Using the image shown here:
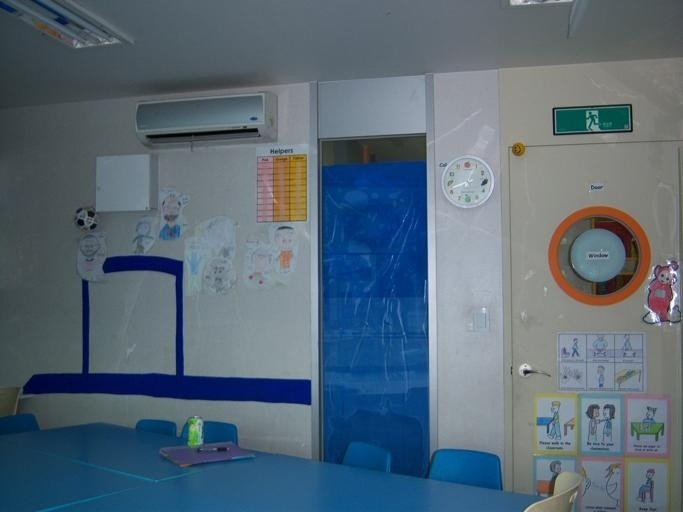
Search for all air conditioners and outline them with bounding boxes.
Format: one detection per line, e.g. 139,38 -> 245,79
134,92 -> 278,153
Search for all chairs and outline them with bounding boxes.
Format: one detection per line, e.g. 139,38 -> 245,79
524,471 -> 583,512
428,447 -> 504,491
341,442 -> 391,473
0,413 -> 41,436
136,418 -> 176,436
181,420 -> 239,447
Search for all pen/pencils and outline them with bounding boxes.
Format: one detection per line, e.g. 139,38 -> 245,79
197,447 -> 231,452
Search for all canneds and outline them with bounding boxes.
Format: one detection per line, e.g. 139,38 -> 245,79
188,416 -> 204,447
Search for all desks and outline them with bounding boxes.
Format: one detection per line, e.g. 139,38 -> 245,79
0,422 -> 549,512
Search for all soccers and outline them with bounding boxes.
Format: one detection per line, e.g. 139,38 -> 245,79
74,206 -> 100,234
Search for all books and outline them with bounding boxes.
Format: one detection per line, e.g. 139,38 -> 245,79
159,441 -> 257,468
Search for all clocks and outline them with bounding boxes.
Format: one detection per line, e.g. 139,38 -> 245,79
441,155 -> 495,209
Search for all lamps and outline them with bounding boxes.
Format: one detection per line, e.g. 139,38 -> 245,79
0,0 -> 136,49
